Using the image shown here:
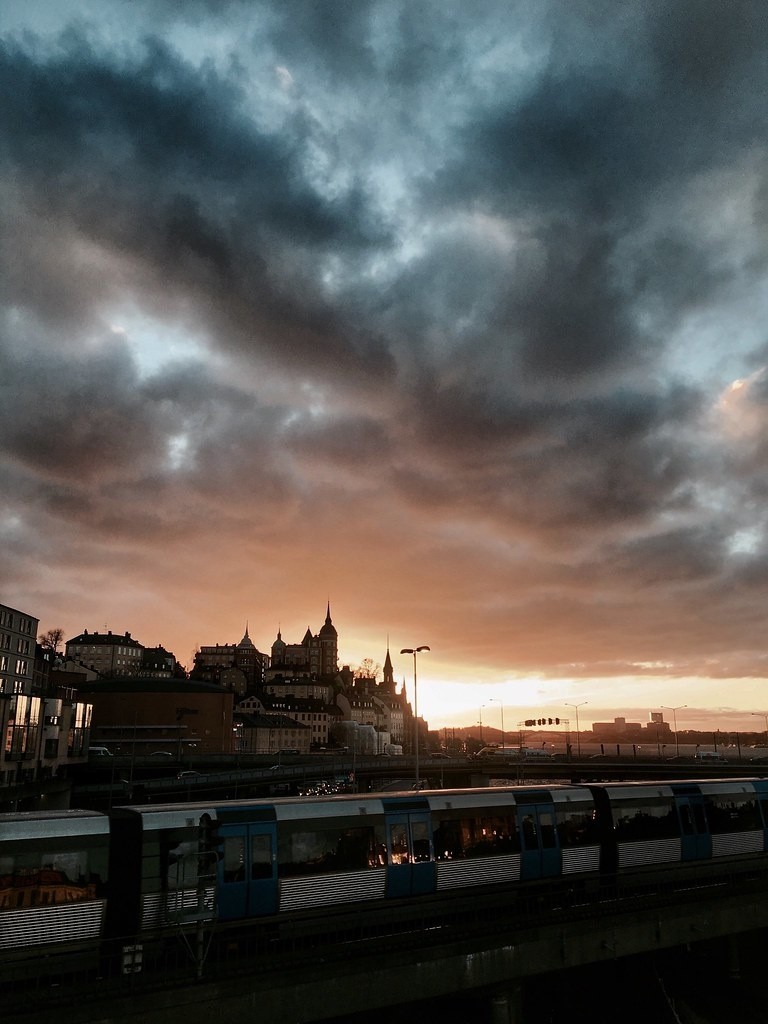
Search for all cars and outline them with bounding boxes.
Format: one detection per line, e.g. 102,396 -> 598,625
750,756 -> 768,763
430,753 -> 450,758
590,753 -> 606,758
177,771 -> 206,779
298,781 -> 352,796
666,756 -> 689,763
151,750 -> 172,756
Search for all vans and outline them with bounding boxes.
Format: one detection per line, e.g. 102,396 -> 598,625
477,747 -> 515,755
273,749 -> 299,755
89,746 -> 113,756
697,751 -> 728,765
525,750 -> 551,757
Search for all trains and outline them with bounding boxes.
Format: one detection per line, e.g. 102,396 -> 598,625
0,777 -> 768,969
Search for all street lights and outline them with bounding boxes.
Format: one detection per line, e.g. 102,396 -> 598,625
401,646 -> 430,790
660,704 -> 687,756
565,702 -> 588,757
490,698 -> 505,760
479,704 -> 486,742
